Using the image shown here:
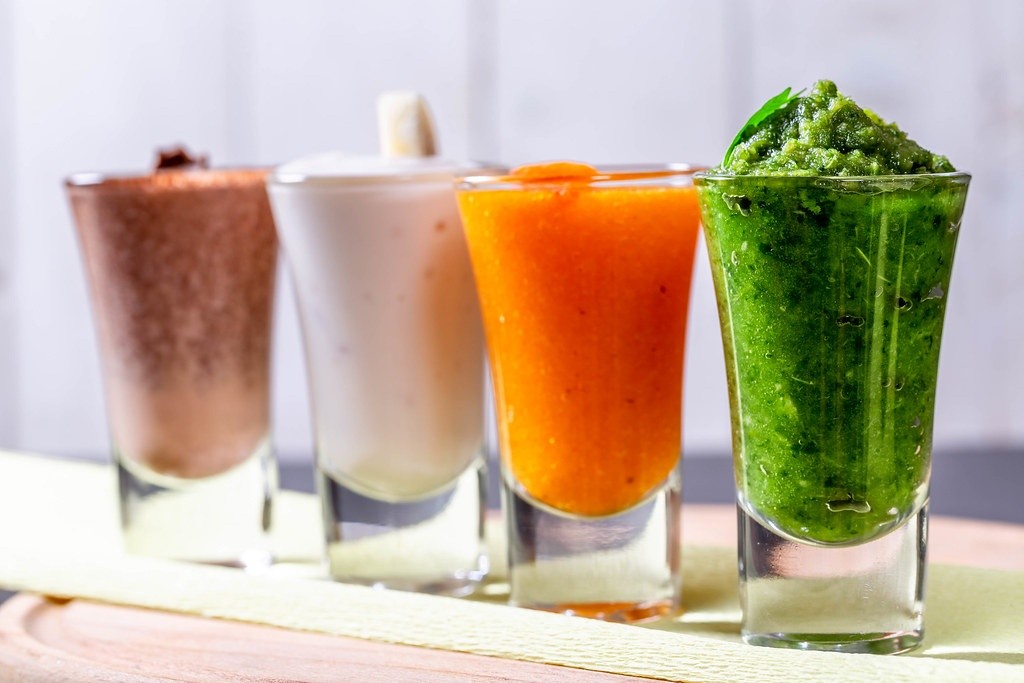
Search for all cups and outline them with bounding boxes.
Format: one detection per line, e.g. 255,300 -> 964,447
62,165 -> 279,564
453,164 -> 707,624
693,165 -> 973,656
265,163 -> 510,596
